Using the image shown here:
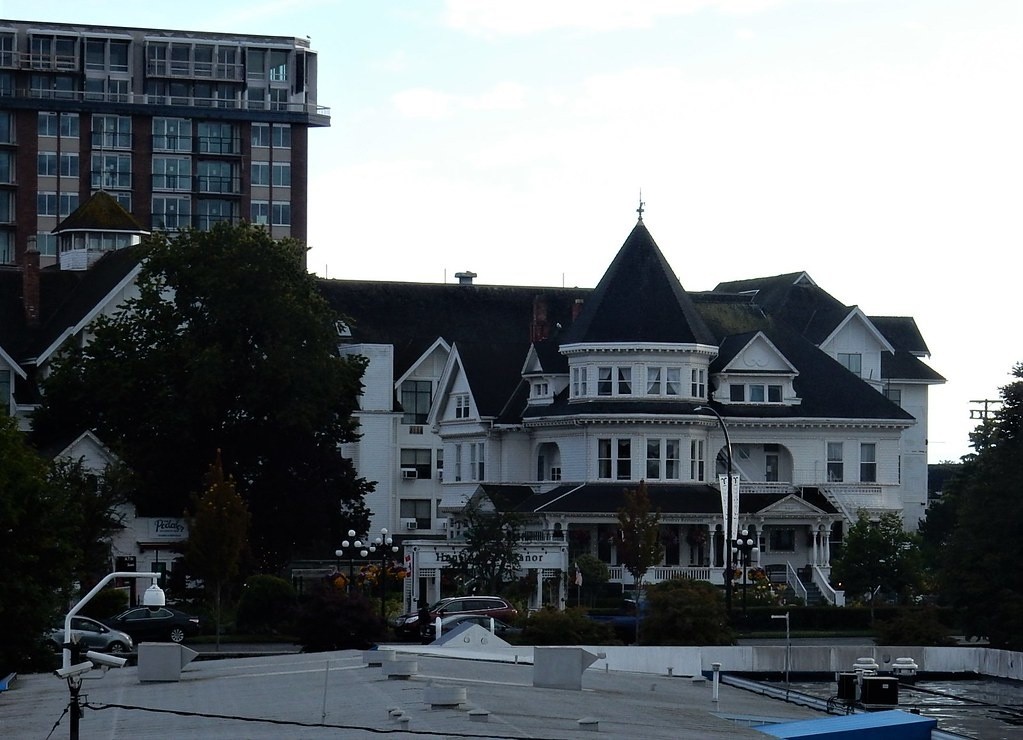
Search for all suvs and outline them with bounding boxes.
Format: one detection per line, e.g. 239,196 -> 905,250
394,596 -> 520,639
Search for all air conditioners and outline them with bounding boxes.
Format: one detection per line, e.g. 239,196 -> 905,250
401,469 -> 418,479
406,521 -> 417,530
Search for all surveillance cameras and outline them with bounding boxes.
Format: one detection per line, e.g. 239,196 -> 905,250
144,588 -> 166,613
53,661 -> 93,680
86,651 -> 128,669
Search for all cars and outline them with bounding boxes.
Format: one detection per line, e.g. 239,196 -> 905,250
104,606 -> 201,644
44,614 -> 134,655
421,614 -> 525,645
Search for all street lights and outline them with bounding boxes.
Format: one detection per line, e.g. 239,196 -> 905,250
62,571 -> 166,740
336,530 -> 370,593
367,528 -> 399,625
737,529 -> 754,621
694,406 -> 733,617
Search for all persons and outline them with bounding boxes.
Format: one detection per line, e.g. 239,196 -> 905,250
368,639 -> 381,667
69,632 -> 80,667
417,602 -> 432,642
805,561 -> 812,583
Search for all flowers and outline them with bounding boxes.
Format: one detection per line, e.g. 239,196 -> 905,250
662,529 -> 680,545
355,563 -> 386,587
388,563 -> 411,583
686,528 -> 708,546
722,568 -> 742,580
322,570 -> 350,589
748,567 -> 765,581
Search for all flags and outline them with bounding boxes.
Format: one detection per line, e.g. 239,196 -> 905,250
575,563 -> 582,586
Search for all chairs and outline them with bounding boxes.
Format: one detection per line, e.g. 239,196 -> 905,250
798,564 -> 812,582
